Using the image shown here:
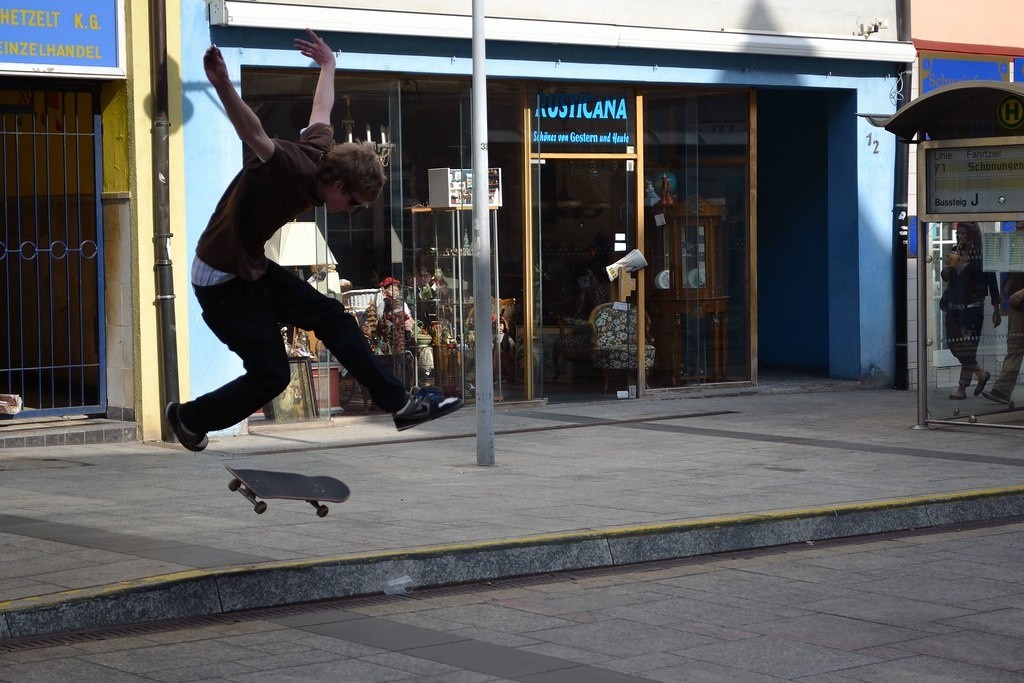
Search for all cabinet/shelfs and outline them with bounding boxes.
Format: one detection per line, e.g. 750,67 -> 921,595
403,203 -> 505,405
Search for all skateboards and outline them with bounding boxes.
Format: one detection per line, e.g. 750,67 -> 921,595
223,463 -> 351,517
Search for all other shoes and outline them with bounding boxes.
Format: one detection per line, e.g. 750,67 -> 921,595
939,288 -> 948,312
974,371 -> 991,395
949,392 -> 967,400
982,390 -> 1011,403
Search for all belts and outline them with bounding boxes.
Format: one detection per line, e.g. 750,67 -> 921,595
948,302 -> 983,310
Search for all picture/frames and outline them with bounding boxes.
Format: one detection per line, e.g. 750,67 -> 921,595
267,356 -> 320,425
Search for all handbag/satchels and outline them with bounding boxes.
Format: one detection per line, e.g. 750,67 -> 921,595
1002,272 -> 1024,297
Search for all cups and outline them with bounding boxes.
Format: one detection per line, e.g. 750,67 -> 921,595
948,250 -> 960,268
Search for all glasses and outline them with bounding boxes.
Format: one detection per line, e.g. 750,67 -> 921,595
347,193 -> 364,208
956,231 -> 967,235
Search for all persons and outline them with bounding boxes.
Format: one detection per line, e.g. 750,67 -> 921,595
982,221 -> 1024,404
377,277 -> 412,350
163,28 -> 464,452
939,221 -> 1001,399
405,261 -> 438,326
491,316 -> 517,384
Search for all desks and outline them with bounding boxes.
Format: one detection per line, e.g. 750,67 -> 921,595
515,322 -> 591,385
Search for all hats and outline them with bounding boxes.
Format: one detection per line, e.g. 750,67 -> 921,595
379,277 -> 400,290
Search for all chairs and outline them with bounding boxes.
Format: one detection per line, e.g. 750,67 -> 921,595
587,301 -> 657,392
456,298 -> 518,381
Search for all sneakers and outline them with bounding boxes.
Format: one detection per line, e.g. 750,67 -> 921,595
163,401 -> 209,452
392,390 -> 465,432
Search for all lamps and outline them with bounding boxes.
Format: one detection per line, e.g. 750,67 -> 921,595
263,217 -> 338,354
